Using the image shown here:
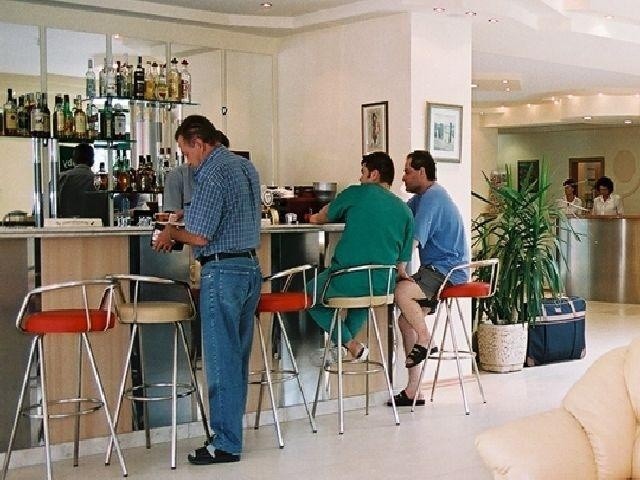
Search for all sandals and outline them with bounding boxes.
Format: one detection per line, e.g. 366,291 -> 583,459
188,444 -> 242,465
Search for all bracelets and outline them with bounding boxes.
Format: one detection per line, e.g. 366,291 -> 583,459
170,225 -> 180,245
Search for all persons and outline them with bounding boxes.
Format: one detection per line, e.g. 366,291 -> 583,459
302,150 -> 416,367
57,144 -> 109,219
152,110 -> 263,466
555,179 -> 584,218
590,176 -> 624,217
161,129 -> 233,216
387,150 -> 471,408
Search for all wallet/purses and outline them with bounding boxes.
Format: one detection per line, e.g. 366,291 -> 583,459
150,222 -> 185,251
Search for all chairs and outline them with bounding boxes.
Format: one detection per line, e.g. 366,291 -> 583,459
1,275 -> 133,480
102,273 -> 209,470
308,261 -> 406,435
246,262 -> 318,450
411,258 -> 500,416
470,337 -> 639,479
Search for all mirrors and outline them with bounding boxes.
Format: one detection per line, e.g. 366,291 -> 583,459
0,22 -> 41,229
42,28 -> 109,221
225,51 -> 276,185
109,34 -> 169,229
169,43 -> 225,172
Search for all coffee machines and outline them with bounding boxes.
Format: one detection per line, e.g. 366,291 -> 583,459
312,182 -> 336,206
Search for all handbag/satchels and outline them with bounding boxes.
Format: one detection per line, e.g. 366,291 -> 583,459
524,296 -> 586,367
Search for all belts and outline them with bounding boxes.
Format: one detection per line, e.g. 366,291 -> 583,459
195,248 -> 257,266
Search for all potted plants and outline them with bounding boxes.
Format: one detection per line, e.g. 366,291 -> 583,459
468,153 -> 597,373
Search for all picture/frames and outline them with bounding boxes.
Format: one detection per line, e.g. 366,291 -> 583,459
361,99 -> 389,158
424,101 -> 463,165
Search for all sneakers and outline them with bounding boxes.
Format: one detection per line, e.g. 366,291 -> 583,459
352,342 -> 370,363
310,345 -> 347,367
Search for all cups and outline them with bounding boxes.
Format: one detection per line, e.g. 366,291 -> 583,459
138,218 -> 150,230
117,217 -> 127,228
94,175 -> 102,191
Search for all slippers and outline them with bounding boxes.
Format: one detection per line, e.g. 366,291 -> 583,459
406,342 -> 438,367
388,390 -> 425,406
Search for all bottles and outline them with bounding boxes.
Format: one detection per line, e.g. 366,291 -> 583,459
97,148 -> 175,192
123,198 -> 132,228
4,88 -> 128,139
84,54 -> 193,102
